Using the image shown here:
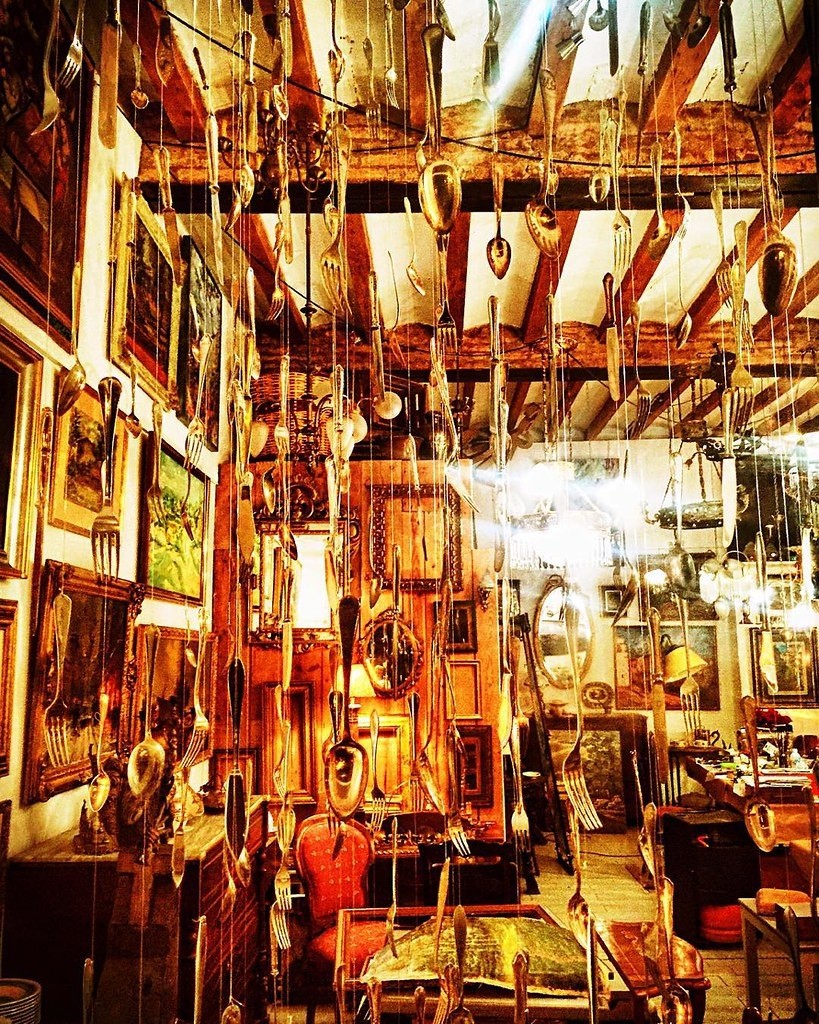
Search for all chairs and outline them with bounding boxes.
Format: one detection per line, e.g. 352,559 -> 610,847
736,786 -> 819,1024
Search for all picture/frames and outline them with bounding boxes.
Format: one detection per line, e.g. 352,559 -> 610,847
18,558 -> 138,805
611,621 -> 721,714
0,0 -> 97,355
534,716 -> 637,830
136,429 -> 211,606
433,600 -> 479,654
749,626 -> 819,710
109,180 -> 185,412
179,235 -> 225,452
247,518 -> 344,642
0,317 -> 44,581
47,367 -> 133,547
370,483 -> 464,595
135,623 -> 215,770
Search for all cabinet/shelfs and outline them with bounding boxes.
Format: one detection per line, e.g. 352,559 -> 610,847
6,794 -> 269,1023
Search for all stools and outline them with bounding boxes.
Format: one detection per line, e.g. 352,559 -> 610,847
596,918 -> 712,1024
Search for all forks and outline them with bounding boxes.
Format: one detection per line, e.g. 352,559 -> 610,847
0,1 -> 818,1024
